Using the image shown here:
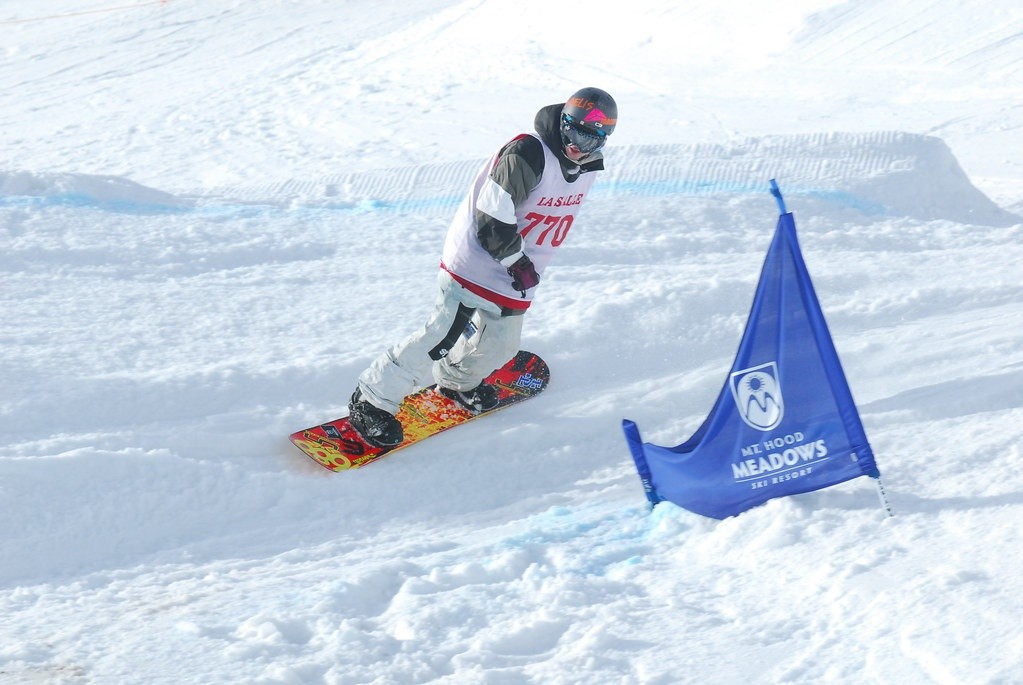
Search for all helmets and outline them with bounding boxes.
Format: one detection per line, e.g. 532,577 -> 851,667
559,87 -> 617,138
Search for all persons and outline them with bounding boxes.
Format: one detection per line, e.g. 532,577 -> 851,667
347,87 -> 618,447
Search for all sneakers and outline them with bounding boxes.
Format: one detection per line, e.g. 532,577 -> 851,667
348,386 -> 403,448
439,378 -> 498,410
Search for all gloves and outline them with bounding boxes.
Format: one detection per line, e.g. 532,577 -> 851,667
507,254 -> 539,292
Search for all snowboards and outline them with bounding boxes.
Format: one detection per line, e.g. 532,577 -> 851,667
289,349 -> 551,475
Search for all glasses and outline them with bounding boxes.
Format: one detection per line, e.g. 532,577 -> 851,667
561,125 -> 603,153
